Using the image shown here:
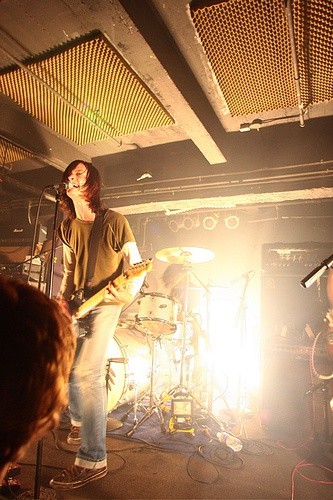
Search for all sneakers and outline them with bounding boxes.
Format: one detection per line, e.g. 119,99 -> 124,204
49,465 -> 108,490
67,425 -> 81,444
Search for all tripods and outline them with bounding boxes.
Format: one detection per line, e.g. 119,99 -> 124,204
118,262 -> 225,444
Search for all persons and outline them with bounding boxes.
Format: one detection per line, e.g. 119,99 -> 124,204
326,267 -> 333,360
49,160 -> 147,491
161,264 -> 200,399
0,277 -> 70,500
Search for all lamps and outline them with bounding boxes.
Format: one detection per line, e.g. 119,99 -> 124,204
172,399 -> 193,416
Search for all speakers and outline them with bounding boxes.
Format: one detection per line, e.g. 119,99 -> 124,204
259,242 -> 333,445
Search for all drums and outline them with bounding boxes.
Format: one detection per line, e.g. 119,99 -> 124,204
136,292 -> 181,336
167,321 -> 194,342
103,318 -> 160,414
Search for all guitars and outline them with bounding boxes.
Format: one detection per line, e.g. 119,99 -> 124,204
61,258 -> 154,340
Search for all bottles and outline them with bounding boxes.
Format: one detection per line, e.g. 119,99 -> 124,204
216,432 -> 243,451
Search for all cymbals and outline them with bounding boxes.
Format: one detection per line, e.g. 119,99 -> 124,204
188,285 -> 231,292
155,246 -> 216,264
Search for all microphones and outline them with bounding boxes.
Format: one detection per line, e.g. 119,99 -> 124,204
304,380 -> 329,397
230,270 -> 254,284
46,182 -> 72,190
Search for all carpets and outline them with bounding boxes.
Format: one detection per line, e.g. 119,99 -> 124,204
106,404 -> 228,458
